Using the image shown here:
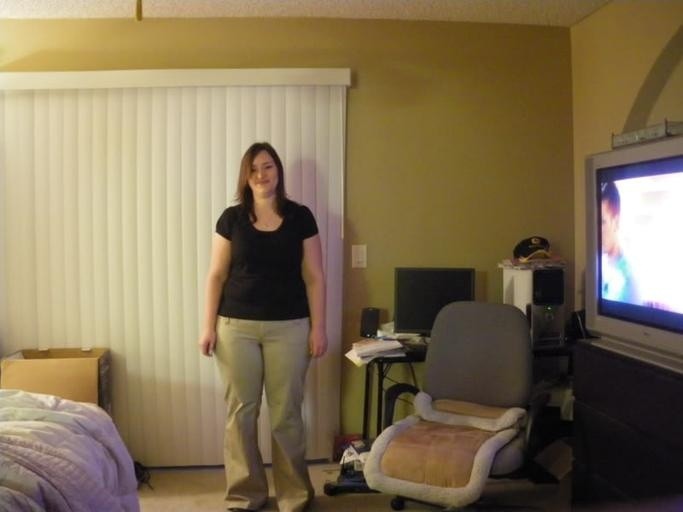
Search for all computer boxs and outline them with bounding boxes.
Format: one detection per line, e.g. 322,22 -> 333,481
502,264 -> 565,350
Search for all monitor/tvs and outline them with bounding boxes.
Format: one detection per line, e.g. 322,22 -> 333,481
393,267 -> 475,345
586,135 -> 682,376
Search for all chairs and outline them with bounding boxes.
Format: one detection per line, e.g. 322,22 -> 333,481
360,301 -> 533,511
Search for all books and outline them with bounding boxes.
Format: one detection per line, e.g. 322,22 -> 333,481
343,338 -> 407,368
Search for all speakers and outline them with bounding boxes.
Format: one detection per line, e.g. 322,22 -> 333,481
359,307 -> 380,338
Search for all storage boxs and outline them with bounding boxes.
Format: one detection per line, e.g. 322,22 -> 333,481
0,345 -> 112,414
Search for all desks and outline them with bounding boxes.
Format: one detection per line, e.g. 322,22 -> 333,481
359,343 -> 576,449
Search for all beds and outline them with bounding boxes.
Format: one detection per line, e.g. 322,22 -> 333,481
0,389 -> 140,512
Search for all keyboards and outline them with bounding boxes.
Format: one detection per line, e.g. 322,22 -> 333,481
405,346 -> 428,354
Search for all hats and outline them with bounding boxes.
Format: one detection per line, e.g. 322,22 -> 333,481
512,236 -> 552,263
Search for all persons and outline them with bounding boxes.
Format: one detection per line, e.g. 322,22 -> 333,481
599,179 -> 644,308
198,141 -> 329,511
